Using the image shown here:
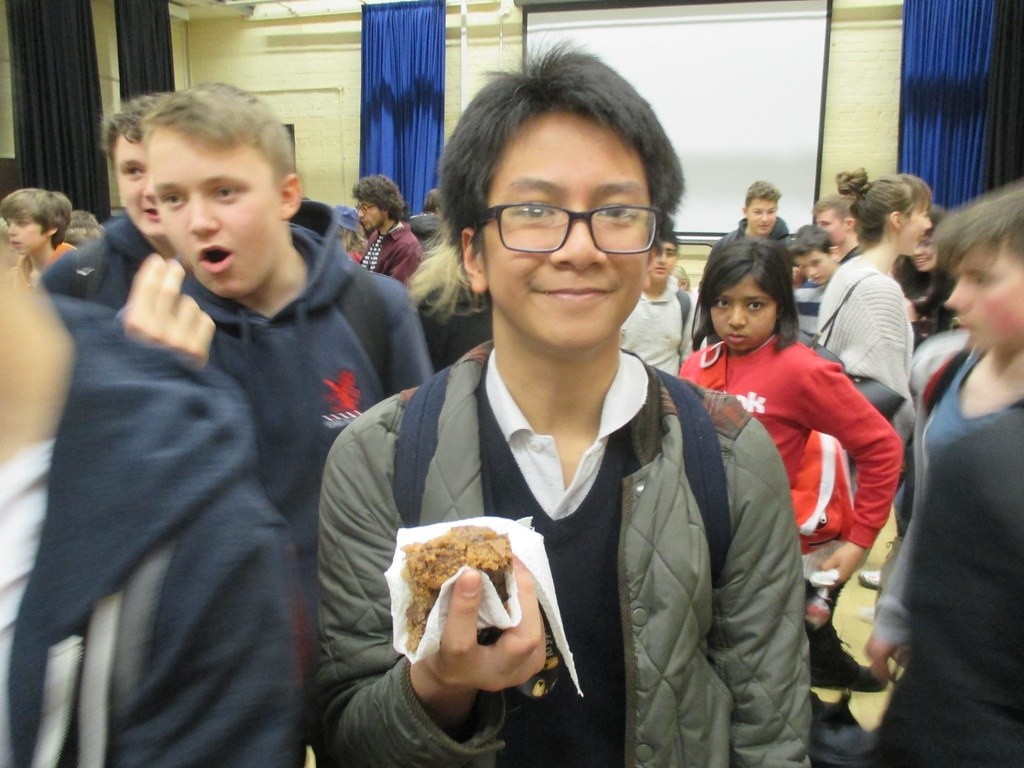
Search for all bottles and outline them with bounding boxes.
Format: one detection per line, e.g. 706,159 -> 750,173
804,585 -> 832,633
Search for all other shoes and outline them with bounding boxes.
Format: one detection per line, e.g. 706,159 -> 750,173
857,569 -> 881,588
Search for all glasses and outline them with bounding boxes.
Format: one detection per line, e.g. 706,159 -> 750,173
469,201 -> 662,254
355,202 -> 376,214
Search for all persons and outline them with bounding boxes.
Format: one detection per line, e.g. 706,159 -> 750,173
0,46 -> 1024,768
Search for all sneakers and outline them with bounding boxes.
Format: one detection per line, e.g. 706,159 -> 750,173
803,618 -> 886,692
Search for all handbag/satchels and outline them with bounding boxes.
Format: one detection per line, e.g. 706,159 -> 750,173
796,272 -> 907,475
